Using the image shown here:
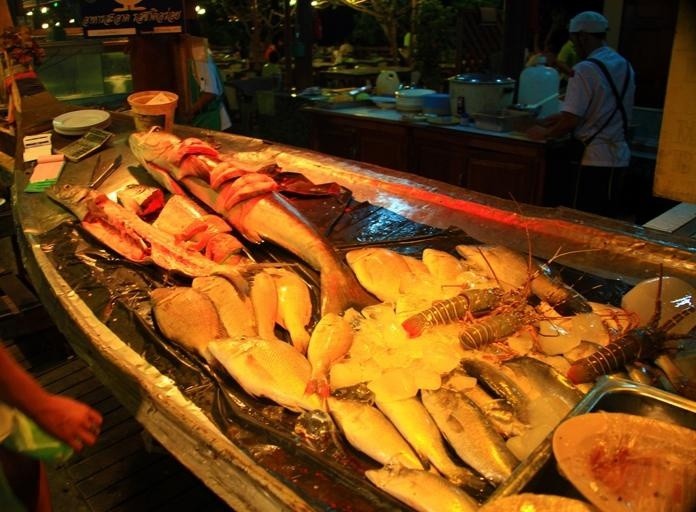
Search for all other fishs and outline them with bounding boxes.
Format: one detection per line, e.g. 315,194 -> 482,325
148,242 -> 690,511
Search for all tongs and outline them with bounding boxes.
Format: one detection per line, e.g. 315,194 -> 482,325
87,151 -> 123,191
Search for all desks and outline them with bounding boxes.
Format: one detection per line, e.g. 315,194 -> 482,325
301,102 -> 574,207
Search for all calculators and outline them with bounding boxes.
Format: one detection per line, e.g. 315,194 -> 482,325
56,127 -> 112,163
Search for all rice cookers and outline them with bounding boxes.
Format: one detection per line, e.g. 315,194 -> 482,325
449,74 -> 517,115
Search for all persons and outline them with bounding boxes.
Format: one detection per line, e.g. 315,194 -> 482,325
0,336 -> 104,512
176,36 -> 232,133
233,11 -> 340,123
523,3 -> 636,215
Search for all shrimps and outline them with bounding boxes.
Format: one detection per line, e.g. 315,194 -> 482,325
402,189 -> 604,357
565,262 -> 696,385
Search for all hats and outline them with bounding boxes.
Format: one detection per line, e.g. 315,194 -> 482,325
569,12 -> 610,35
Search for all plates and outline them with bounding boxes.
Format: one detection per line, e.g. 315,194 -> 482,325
369,94 -> 395,108
421,93 -> 450,115
54,109 -> 113,136
393,88 -> 434,113
